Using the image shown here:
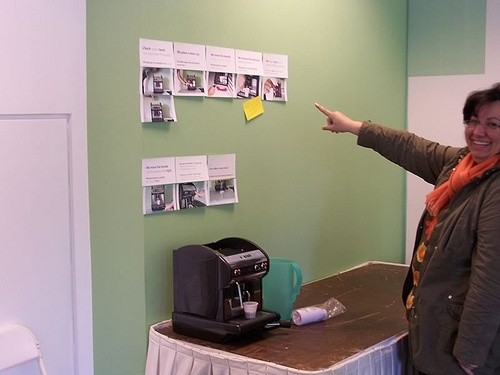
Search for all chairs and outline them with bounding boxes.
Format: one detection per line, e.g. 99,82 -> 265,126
0,324 -> 48,375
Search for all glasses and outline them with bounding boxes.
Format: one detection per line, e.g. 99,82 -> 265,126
463,120 -> 500,129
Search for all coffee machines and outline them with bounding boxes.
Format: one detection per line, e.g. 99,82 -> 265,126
172,237 -> 282,339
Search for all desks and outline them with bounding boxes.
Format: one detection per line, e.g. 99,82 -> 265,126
145,261 -> 410,375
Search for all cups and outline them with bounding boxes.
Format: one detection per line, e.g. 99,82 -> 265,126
243,302 -> 258,319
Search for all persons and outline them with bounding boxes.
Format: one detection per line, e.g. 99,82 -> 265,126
313,82 -> 500,375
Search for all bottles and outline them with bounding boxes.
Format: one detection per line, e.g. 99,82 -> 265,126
292,306 -> 329,326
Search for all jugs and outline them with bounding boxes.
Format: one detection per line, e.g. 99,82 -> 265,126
262,257 -> 303,322
